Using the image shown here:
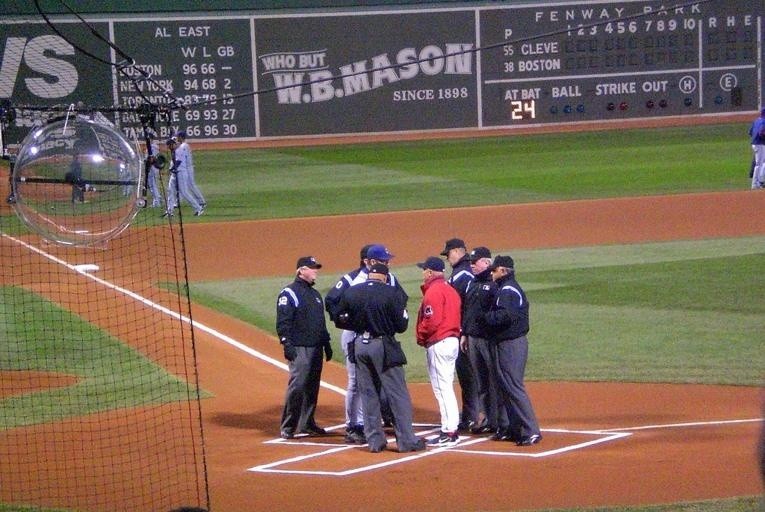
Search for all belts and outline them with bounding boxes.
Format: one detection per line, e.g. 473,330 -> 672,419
359,333 -> 384,340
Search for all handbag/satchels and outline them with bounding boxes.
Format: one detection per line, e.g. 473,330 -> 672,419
382,333 -> 408,369
347,337 -> 359,363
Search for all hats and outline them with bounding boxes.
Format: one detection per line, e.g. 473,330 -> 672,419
360,245 -> 373,262
297,255 -> 322,269
467,246 -> 491,263
148,131 -> 186,145
488,255 -> 515,271
416,257 -> 446,274
368,243 -> 395,262
150,201 -> 207,218
440,237 -> 466,257
371,264 -> 388,276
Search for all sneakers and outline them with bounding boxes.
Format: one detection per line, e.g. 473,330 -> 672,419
279,426 -> 327,440
344,420 -> 426,453
426,430 -> 460,448
459,419 -> 543,447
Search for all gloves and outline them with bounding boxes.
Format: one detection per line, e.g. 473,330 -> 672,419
284,339 -> 297,362
323,341 -> 334,362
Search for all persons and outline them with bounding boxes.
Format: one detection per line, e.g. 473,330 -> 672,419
71,153 -> 87,204
747,108 -> 765,190
119,131 -> 207,219
